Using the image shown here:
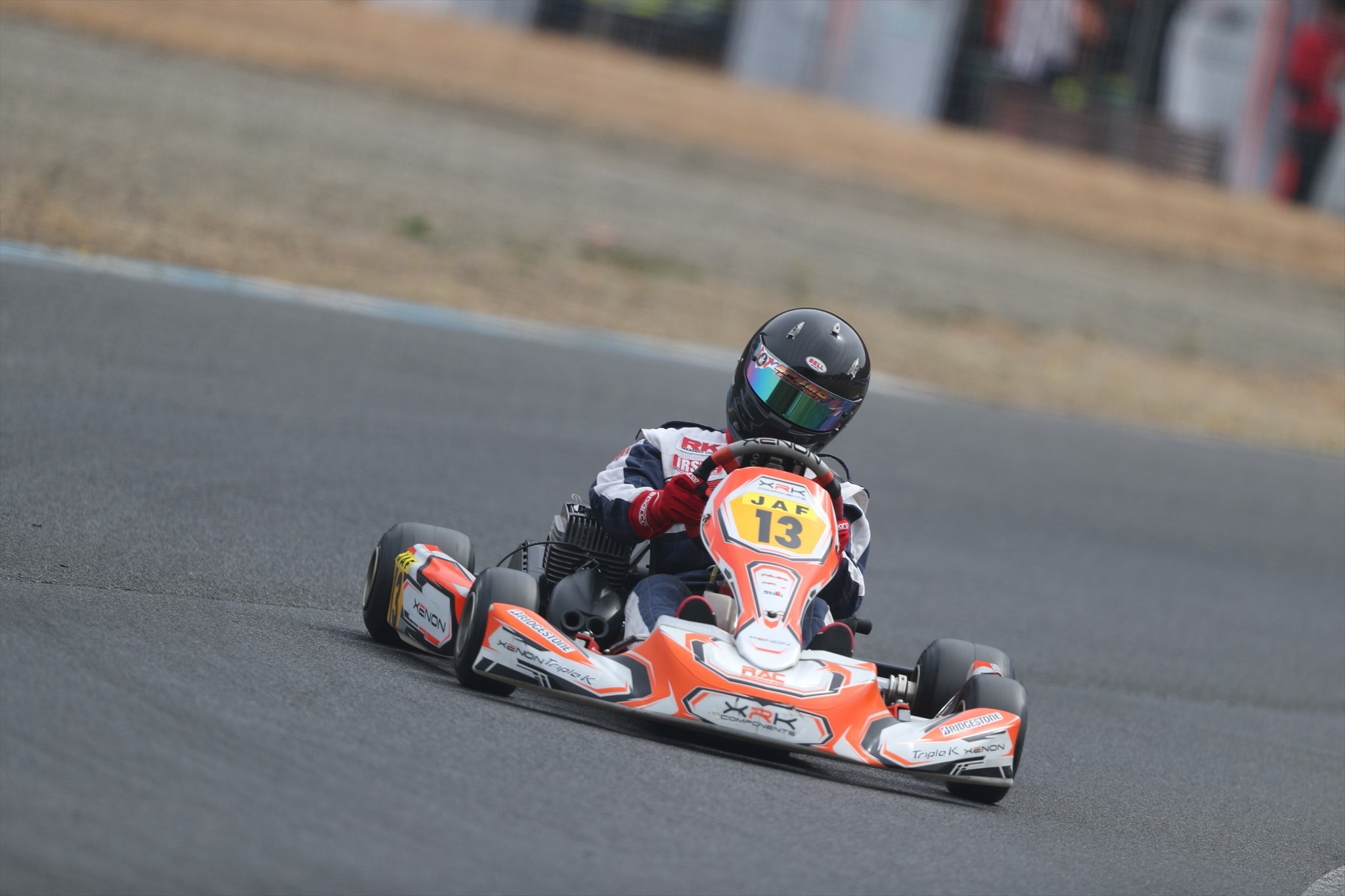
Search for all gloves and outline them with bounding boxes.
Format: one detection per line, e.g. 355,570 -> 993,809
836,519 -> 850,555
628,471 -> 709,538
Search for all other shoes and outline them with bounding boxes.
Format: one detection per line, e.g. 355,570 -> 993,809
806,620 -> 854,658
677,595 -> 718,626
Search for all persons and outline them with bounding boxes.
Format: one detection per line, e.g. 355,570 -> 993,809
591,307 -> 871,658
1270,0 -> 1345,207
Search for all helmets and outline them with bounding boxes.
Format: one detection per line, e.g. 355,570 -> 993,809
726,308 -> 871,464
